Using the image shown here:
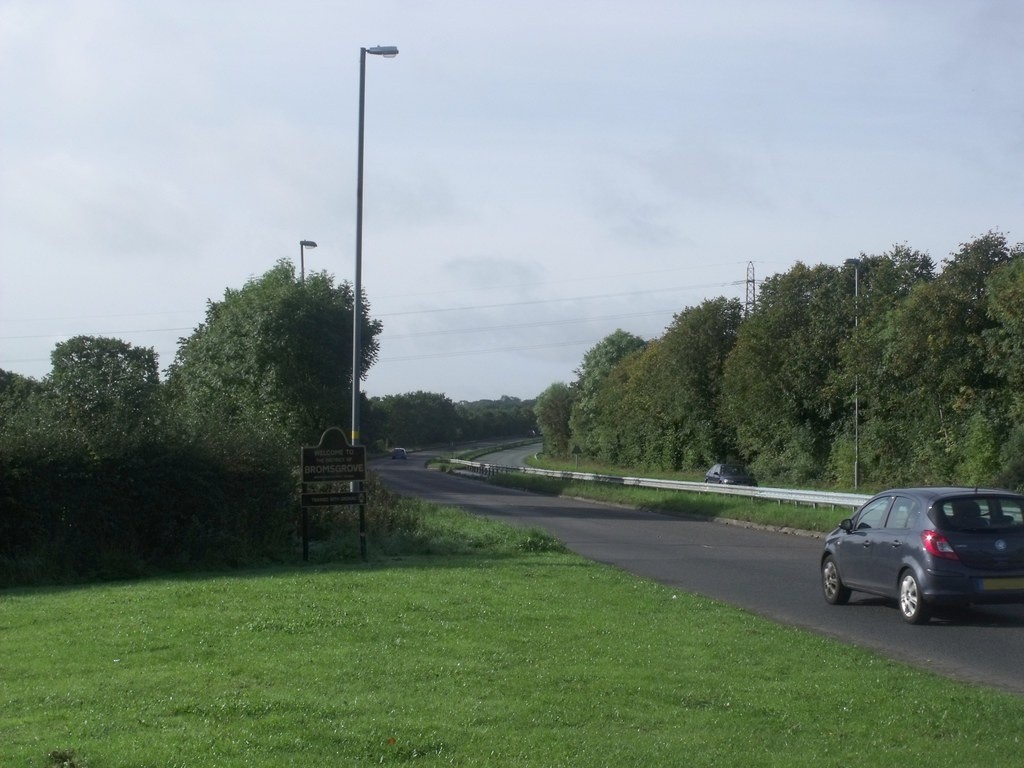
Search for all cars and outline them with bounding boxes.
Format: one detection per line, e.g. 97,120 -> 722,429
820,487 -> 1024,625
392,448 -> 406,459
704,463 -> 758,487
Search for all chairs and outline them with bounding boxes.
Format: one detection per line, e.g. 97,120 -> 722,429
960,502 -> 988,525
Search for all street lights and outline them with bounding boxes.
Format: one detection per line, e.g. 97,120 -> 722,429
843,258 -> 859,489
350,46 -> 399,493
300,240 -> 317,287
